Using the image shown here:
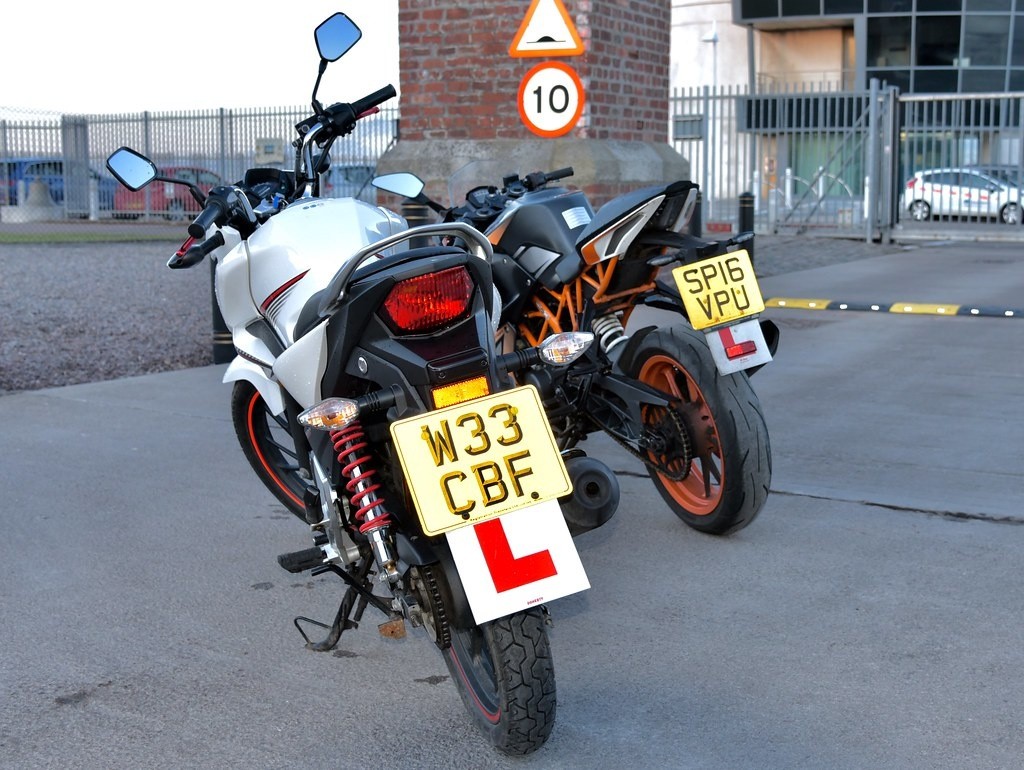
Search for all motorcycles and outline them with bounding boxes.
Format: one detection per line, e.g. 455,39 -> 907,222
371,167 -> 781,536
105,10 -> 621,757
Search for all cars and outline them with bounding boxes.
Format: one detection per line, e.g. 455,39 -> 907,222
902,168 -> 1024,224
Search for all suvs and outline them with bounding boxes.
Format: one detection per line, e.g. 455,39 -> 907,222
0,155 -> 116,218
113,166 -> 227,220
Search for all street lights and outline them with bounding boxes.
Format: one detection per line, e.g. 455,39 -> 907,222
701,30 -> 717,206
